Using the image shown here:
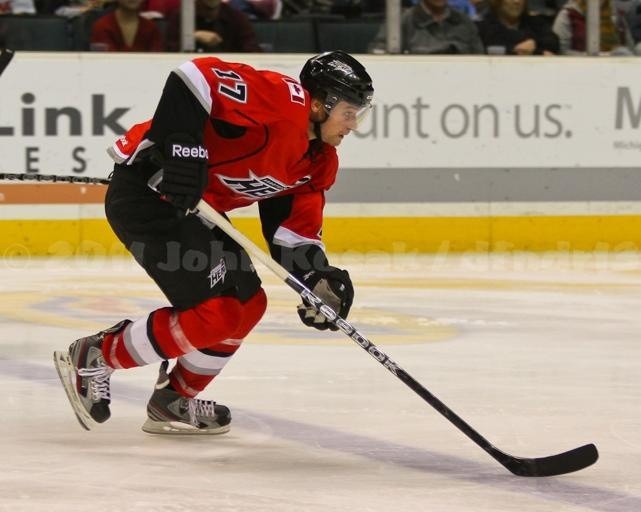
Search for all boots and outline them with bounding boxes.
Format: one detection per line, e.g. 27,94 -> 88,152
67,319 -> 132,425
145,361 -> 230,431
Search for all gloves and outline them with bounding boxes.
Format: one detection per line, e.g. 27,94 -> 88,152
291,243 -> 354,332
161,134 -> 209,208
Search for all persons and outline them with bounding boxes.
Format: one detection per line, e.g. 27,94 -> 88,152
367,1 -> 641,57
68,50 -> 374,430
1,2 -> 298,55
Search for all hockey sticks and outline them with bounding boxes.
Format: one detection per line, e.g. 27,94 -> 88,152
148,166 -> 598,477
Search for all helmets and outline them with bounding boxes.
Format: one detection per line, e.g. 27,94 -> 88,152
299,49 -> 373,129
